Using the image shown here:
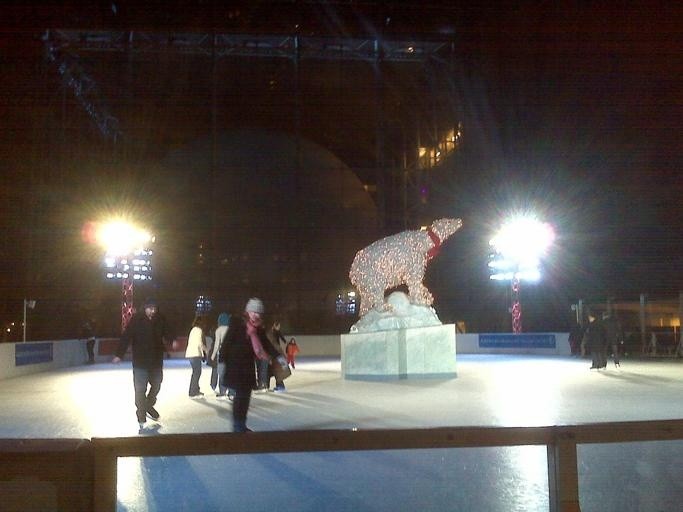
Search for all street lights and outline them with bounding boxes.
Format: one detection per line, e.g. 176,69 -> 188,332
488,209 -> 555,333
95,210 -> 153,339
22,298 -> 36,342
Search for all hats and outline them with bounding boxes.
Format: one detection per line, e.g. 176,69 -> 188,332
143,298 -> 158,308
245,296 -> 266,316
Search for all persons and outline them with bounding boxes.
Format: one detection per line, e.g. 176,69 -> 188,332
183,314 -> 208,399
217,295 -> 266,433
284,337 -> 300,370
209,312 -> 291,404
567,308 -> 624,370
81,321 -> 96,364
110,298 -> 170,424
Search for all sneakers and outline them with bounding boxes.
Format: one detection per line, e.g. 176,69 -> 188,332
146,407 -> 160,419
137,415 -> 148,424
189,383 -> 287,396
